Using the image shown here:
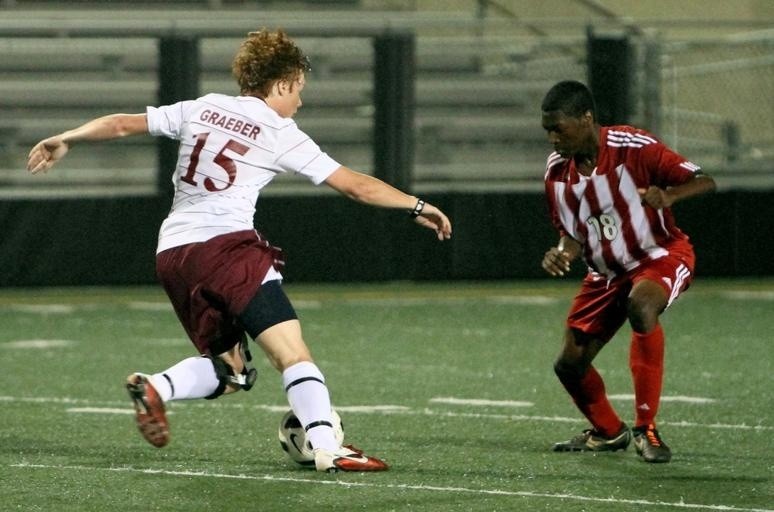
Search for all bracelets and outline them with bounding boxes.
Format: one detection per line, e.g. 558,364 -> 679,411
408,199 -> 425,219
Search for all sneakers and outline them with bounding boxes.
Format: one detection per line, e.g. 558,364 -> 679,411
125,372 -> 169,448
632,425 -> 671,463
552,421 -> 631,453
310,443 -> 388,472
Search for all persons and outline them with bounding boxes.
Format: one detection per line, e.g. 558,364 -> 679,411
541,81 -> 715,464
25,28 -> 452,473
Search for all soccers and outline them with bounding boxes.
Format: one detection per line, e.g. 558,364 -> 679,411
278,406 -> 343,463
